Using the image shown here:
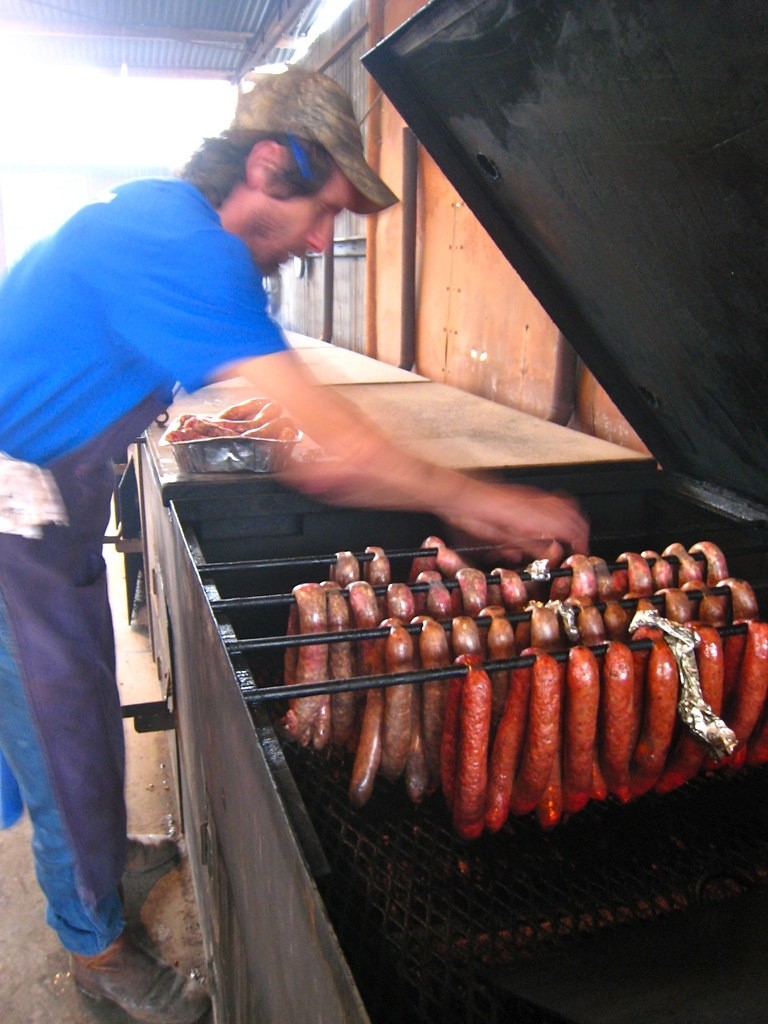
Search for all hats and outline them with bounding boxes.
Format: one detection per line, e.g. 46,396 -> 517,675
232,58 -> 397,217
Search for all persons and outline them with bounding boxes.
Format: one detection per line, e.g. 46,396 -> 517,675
0,65 -> 590,1024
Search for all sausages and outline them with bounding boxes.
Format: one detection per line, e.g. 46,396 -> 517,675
282,536 -> 768,837
164,396 -> 300,441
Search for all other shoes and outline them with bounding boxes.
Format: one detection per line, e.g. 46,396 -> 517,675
118,827 -> 180,875
67,928 -> 212,1024
